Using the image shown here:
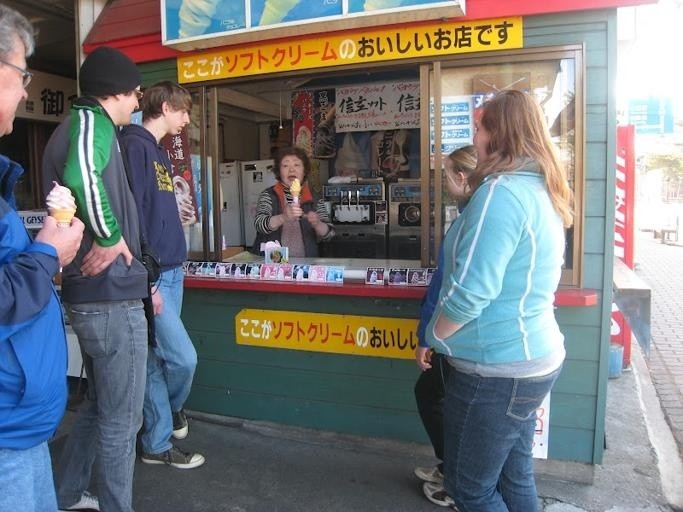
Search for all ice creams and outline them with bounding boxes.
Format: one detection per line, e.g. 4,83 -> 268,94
334,132 -> 368,178
45,180 -> 78,229
289,178 -> 302,205
172,175 -> 197,251
176,0 -> 216,39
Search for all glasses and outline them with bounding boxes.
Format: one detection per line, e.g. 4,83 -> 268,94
0,58 -> 33,88
134,88 -> 143,98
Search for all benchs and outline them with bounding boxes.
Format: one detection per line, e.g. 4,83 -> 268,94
611,255 -> 654,359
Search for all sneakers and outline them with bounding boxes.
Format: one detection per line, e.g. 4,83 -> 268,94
58,491 -> 100,512
423,481 -> 460,511
141,444 -> 205,469
414,466 -> 445,484
171,409 -> 188,439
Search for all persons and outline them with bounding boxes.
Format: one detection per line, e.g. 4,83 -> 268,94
0,1 -> 86,512
409,145 -> 480,512
116,80 -> 204,469
250,146 -> 336,258
40,46 -> 154,512
424,92 -> 574,512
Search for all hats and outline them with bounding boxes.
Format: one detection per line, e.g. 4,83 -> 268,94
80,47 -> 141,96
